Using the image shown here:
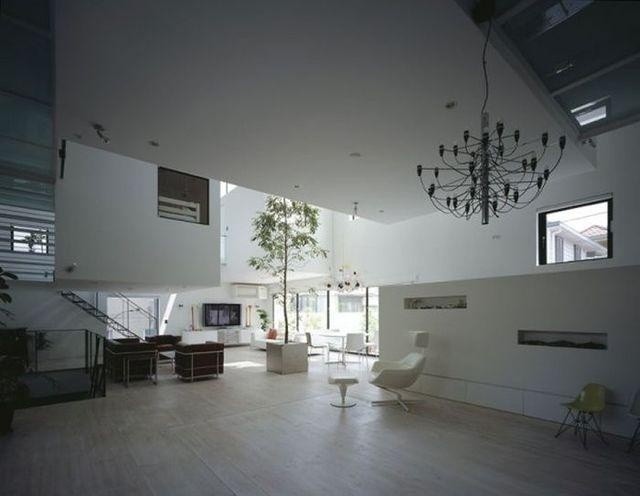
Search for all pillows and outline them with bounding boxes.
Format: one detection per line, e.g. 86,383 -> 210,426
267,328 -> 278,340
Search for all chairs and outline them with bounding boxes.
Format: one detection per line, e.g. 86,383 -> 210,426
554,381 -> 610,451
626,391 -> 640,453
368,332 -> 429,413
304,332 -> 327,359
344,331 -> 375,367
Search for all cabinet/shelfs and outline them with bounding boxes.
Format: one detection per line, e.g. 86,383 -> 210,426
181,326 -> 256,347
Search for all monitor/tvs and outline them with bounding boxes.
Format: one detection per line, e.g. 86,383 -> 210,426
205,304 -> 242,326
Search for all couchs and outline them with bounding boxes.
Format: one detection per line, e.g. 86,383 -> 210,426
144,334 -> 181,359
174,341 -> 224,381
250,330 -> 304,352
103,336 -> 159,388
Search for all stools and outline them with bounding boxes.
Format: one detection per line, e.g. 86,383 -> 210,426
326,375 -> 360,408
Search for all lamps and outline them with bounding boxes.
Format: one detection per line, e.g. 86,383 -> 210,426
93,123 -> 113,144
326,235 -> 361,296
415,0 -> 565,224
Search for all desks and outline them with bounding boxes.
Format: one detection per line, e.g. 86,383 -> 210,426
321,331 -> 351,364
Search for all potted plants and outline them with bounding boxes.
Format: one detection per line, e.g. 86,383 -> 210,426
0,265 -> 54,435
245,193 -> 329,376
